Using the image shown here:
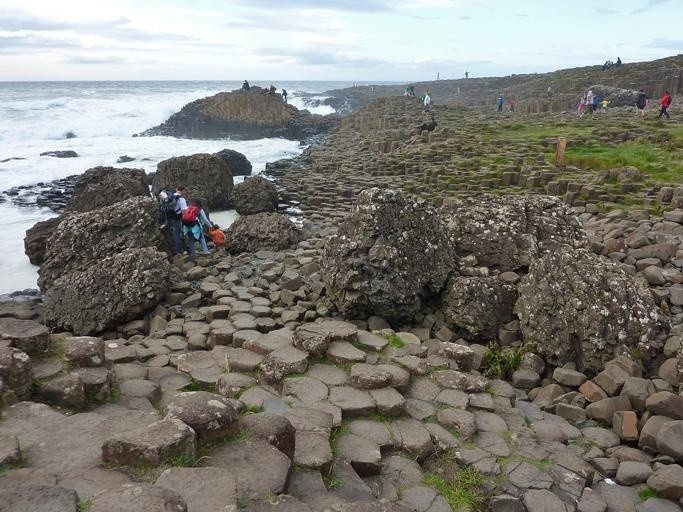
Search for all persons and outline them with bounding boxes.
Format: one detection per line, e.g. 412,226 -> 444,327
416,117 -> 438,135
615,57 -> 621,67
655,91 -> 671,120
240,79 -> 248,89
409,86 -> 416,97
402,85 -> 410,98
269,84 -> 275,93
420,90 -> 428,102
182,197 -> 214,258
281,89 -> 287,101
165,184 -> 186,256
496,96 -> 503,113
421,92 -> 432,116
634,89 -> 648,117
577,86 -> 611,119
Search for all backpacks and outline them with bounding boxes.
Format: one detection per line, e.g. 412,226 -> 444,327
182,203 -> 201,227
165,193 -> 181,220
